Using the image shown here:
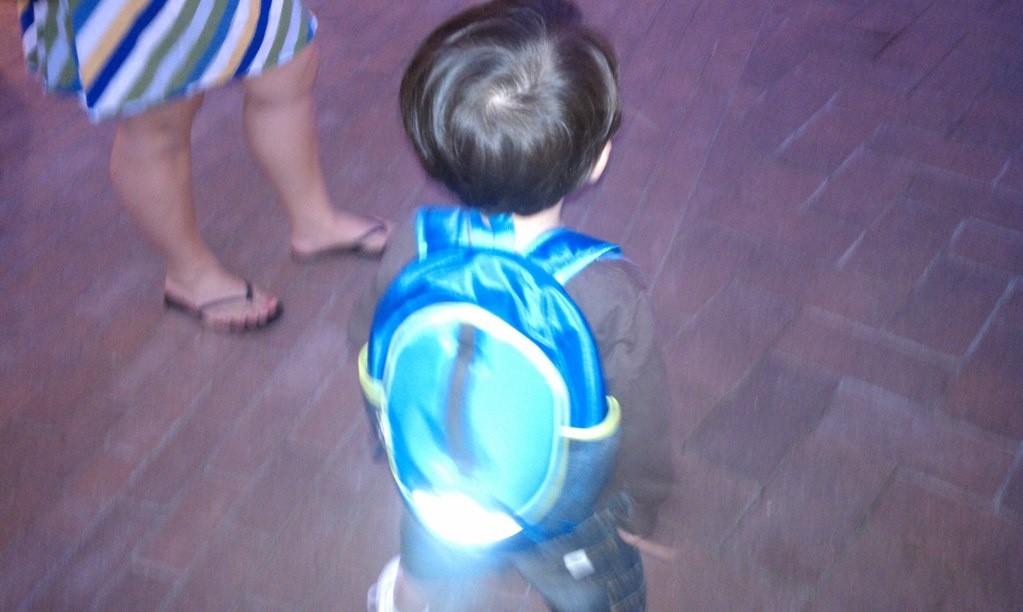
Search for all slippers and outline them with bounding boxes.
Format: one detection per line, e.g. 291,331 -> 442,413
289,218 -> 396,261
165,270 -> 285,331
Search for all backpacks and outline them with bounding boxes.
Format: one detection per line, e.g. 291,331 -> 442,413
358,202 -> 622,551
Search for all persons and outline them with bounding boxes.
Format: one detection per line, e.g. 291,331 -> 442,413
16,0 -> 399,333
356,1 -> 676,612
